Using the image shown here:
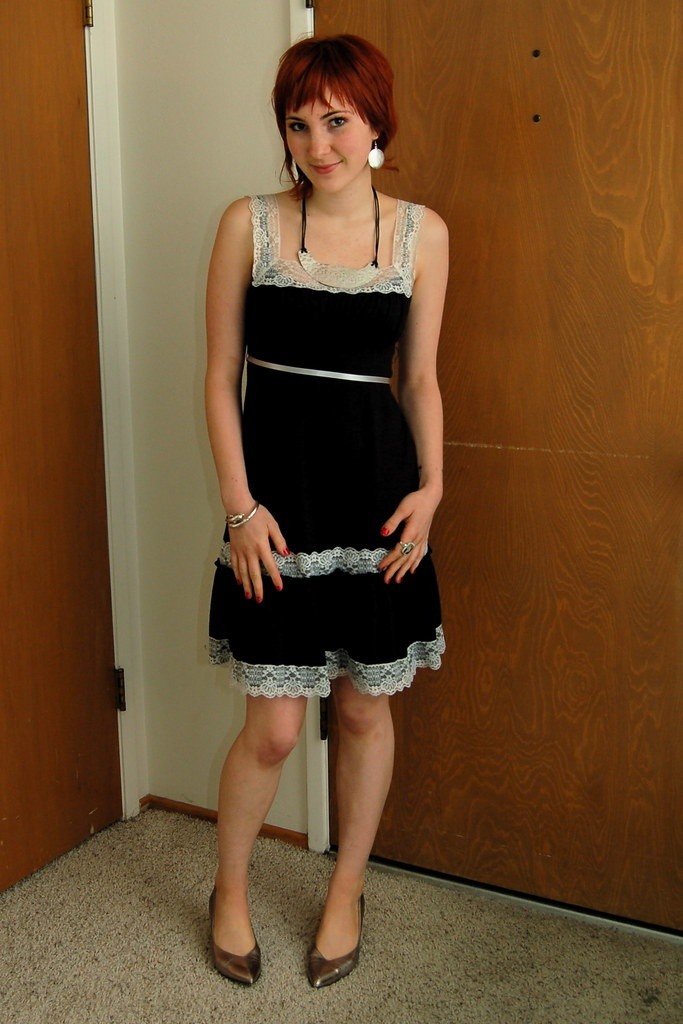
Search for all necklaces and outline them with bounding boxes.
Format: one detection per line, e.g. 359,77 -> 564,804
298,186 -> 381,288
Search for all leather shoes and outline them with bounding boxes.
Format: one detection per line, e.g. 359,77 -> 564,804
307,895 -> 366,989
209,886 -> 261,984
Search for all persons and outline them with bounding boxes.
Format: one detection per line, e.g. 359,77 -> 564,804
204,34 -> 448,985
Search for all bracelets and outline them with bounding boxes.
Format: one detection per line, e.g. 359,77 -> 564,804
225,501 -> 260,528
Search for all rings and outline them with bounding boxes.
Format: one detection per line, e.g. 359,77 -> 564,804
399,540 -> 414,555
